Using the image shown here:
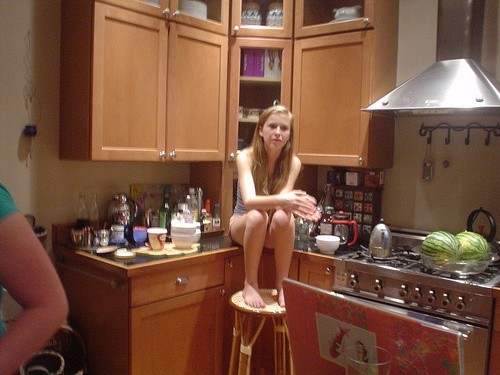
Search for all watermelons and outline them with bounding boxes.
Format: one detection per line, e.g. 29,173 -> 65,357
420,230 -> 463,265
456,231 -> 491,261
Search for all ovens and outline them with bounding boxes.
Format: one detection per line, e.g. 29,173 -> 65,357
281,275 -> 491,375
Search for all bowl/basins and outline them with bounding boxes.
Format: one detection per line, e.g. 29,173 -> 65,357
315,234 -> 341,254
170,222 -> 202,250
333,5 -> 362,20
413,246 -> 499,274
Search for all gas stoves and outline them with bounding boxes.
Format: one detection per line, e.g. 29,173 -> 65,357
333,225 -> 499,327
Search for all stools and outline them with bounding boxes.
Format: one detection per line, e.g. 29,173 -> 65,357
228,289 -> 288,375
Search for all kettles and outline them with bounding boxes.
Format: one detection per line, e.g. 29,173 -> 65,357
467,208 -> 500,265
368,219 -> 394,258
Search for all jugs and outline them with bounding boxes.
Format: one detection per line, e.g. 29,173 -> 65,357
332,210 -> 360,249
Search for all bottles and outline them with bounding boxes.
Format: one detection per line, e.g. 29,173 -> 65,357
159,184 -> 222,234
293,183 -> 336,248
72,190 -> 138,248
242,0 -> 283,26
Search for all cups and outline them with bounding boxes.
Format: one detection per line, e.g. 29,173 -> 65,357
345,344 -> 391,375
238,139 -> 246,153
147,228 -> 168,251
133,225 -> 149,245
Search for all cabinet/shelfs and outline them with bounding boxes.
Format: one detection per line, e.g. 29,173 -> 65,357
53,253 -> 264,375
59,0 -> 399,168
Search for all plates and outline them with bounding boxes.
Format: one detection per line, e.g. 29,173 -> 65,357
178,0 -> 208,19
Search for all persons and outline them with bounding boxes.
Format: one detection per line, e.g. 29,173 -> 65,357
228,105 -> 322,308
0,183 -> 68,375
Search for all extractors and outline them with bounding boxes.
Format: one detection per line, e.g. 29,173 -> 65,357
359,0 -> 500,117
